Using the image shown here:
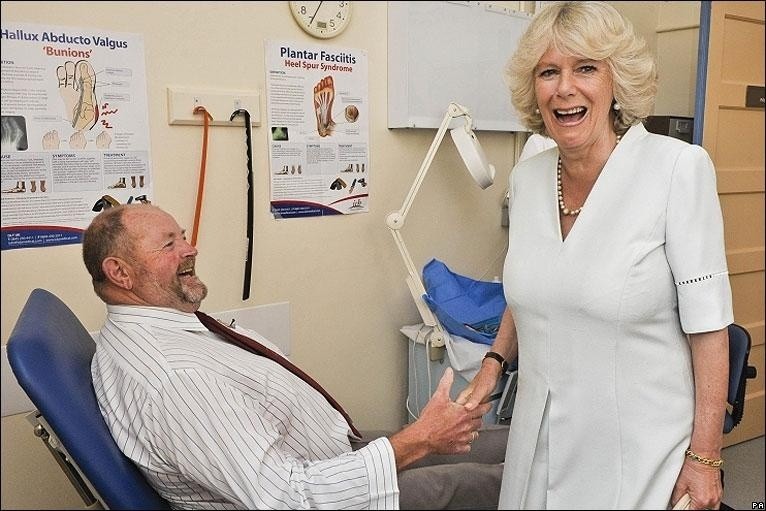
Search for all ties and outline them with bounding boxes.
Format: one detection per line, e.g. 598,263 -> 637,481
194,311 -> 364,439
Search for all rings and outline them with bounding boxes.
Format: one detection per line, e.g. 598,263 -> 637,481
469,431 -> 478,445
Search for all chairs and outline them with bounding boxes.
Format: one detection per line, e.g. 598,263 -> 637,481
718,320 -> 757,511
6,289 -> 188,510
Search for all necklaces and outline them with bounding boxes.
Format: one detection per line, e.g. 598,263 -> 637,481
557,135 -> 621,216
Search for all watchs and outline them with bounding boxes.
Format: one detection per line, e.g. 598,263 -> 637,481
480,351 -> 508,373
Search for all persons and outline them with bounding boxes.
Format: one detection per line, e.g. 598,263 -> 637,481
454,1 -> 736,510
79,200 -> 509,509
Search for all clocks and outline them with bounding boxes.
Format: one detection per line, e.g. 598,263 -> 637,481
289,0 -> 353,39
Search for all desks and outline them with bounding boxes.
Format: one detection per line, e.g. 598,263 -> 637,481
400,323 -> 521,432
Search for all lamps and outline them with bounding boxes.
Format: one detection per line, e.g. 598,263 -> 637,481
385,100 -> 497,349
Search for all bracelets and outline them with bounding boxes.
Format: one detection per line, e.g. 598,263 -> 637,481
686,449 -> 724,467
